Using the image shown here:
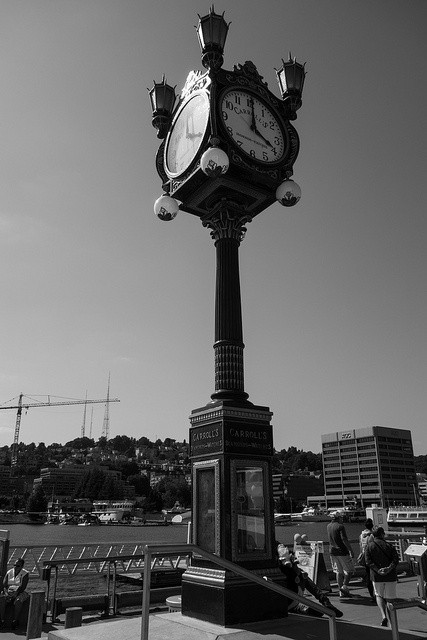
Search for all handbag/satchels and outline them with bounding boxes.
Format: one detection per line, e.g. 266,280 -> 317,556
357,553 -> 365,566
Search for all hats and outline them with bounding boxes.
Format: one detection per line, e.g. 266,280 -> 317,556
372,526 -> 384,537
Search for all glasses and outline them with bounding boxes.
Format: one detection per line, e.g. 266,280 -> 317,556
13,564 -> 22,567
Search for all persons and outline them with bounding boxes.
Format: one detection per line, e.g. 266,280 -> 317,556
365,525 -> 399,627
0,558 -> 28,625
359,518 -> 377,603
327,511 -> 354,598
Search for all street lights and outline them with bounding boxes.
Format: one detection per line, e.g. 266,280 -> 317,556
146,2 -> 307,628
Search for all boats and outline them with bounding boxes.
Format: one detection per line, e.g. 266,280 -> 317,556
43,512 -> 102,527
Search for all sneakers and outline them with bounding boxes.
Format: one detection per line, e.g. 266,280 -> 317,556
381,618 -> 387,626
339,591 -> 353,598
368,596 -> 374,602
340,585 -> 352,598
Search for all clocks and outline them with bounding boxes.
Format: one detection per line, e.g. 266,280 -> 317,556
219,85 -> 290,164
163,90 -> 210,179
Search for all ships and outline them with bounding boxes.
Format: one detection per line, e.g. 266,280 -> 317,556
336,496 -> 365,523
300,504 -> 336,521
386,500 -> 426,527
